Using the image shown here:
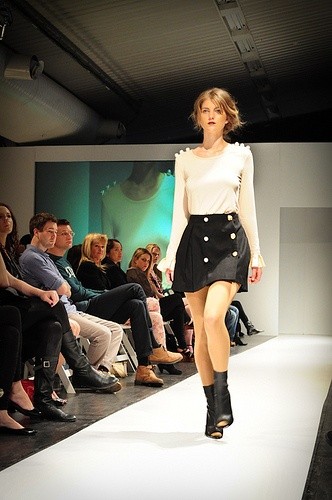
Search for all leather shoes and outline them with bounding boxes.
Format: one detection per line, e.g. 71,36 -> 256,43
2,393 -> 42,418
0,427 -> 37,436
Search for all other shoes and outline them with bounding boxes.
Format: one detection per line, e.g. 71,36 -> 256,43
186,344 -> 194,358
179,342 -> 190,358
98,368 -> 122,392
230,338 -> 235,346
149,344 -> 183,364
134,365 -> 164,386
50,392 -> 68,406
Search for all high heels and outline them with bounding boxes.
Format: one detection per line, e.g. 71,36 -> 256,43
157,364 -> 182,375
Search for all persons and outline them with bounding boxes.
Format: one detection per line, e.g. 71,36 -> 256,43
98,161 -> 175,277
156,87 -> 266,440
0,205 -> 264,435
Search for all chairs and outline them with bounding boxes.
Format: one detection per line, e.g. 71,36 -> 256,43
24,321 -> 180,399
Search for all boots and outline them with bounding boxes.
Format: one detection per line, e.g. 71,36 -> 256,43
203,384 -> 224,439
233,332 -> 247,346
32,356 -> 76,423
213,371 -> 234,430
60,328 -> 118,391
245,321 -> 265,336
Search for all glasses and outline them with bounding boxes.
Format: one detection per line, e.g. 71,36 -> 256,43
57,230 -> 75,239
